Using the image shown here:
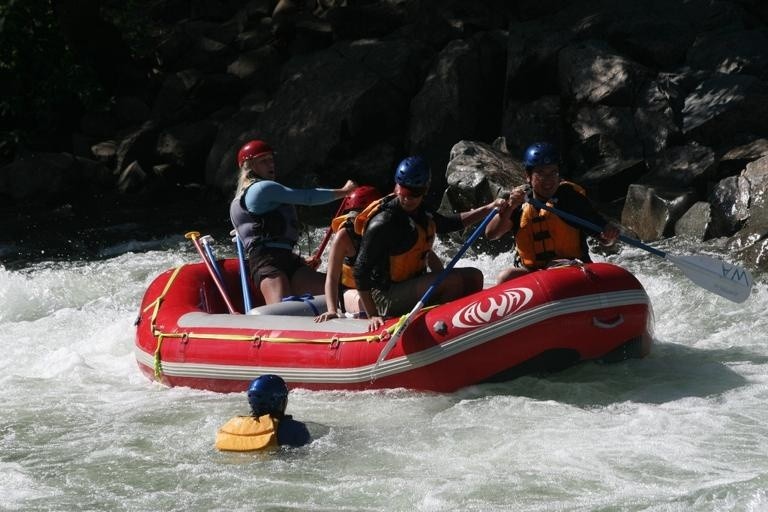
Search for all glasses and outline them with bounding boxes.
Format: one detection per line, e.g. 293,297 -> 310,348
398,184 -> 425,198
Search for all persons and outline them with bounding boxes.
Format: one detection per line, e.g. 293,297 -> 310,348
313,185 -> 380,323
215,374 -> 310,452
484,142 -> 620,285
353,155 -> 508,332
229,139 -> 355,304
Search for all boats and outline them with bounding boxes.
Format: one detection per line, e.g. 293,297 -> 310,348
136,258 -> 650,394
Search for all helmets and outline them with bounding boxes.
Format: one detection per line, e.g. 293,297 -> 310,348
344,186 -> 381,209
237,139 -> 273,168
523,142 -> 564,170
395,155 -> 431,187
248,375 -> 288,415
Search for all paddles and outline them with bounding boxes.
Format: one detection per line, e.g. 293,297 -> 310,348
378,193 -> 508,364
524,197 -> 752,302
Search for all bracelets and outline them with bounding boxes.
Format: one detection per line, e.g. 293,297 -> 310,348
366,310 -> 379,320
